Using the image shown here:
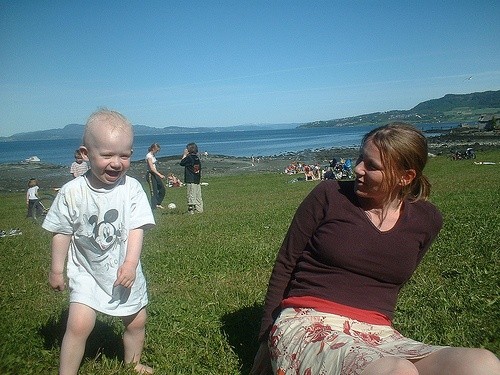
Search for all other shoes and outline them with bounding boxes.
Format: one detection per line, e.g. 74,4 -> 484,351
195,209 -> 200,213
188,207 -> 194,215
156,205 -> 165,209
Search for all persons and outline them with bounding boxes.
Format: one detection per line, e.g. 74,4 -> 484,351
26,177 -> 43,217
179,143 -> 204,214
283,157 -> 354,181
70,149 -> 89,178
437,148 -> 471,160
248,120 -> 500,375
166,173 -> 182,188
250,153 -> 260,167
42,108 -> 155,375
145,143 -> 167,210
204,149 -> 208,158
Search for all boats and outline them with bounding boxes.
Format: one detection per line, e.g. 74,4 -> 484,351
26,155 -> 40,161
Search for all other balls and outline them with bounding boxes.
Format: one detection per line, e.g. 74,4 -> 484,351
168,203 -> 176,209
